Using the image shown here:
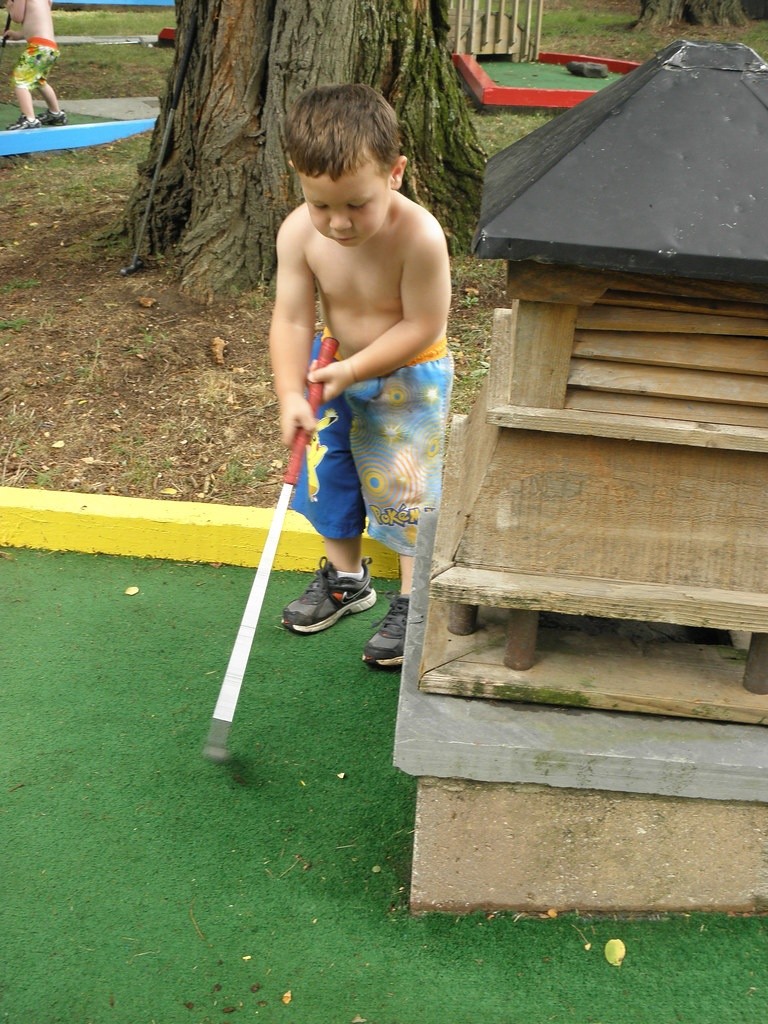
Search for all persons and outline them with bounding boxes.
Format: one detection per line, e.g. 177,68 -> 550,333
268,81 -> 456,668
3,0 -> 68,131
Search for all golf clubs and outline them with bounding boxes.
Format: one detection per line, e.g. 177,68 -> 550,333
201,335 -> 341,764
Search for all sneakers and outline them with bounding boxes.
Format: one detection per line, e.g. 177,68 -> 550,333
35,108 -> 68,126
6,113 -> 42,130
280,556 -> 377,633
362,590 -> 410,665
